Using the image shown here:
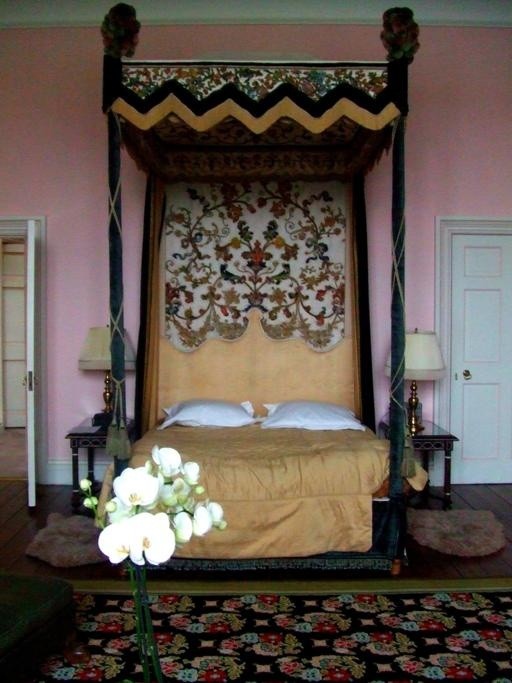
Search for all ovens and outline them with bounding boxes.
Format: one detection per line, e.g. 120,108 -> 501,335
104,424 -> 430,560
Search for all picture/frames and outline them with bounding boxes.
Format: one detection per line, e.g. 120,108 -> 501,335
64,417 -> 138,509
379,420 -> 459,511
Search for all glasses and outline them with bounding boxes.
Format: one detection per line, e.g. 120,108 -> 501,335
383,327 -> 446,433
78,325 -> 137,426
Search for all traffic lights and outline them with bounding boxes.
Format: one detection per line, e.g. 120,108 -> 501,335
156,400 -> 366,432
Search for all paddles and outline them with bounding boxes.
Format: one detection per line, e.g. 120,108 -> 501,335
406,507 -> 506,557
26,591 -> 512,683
26,512 -> 110,567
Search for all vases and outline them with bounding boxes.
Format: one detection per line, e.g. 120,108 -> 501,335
96,444 -> 228,683
